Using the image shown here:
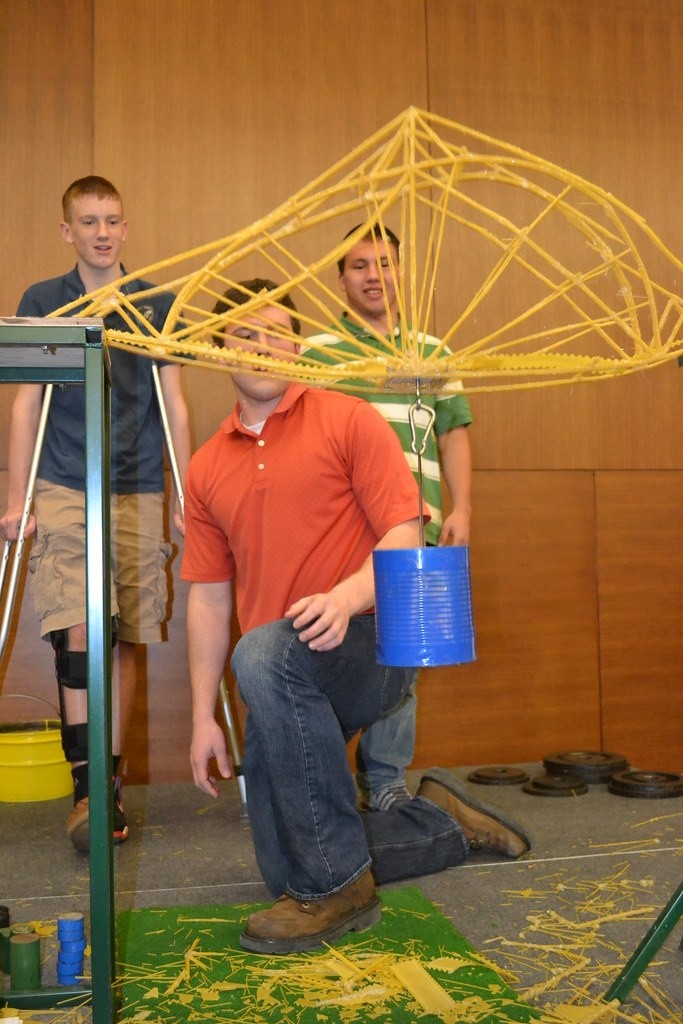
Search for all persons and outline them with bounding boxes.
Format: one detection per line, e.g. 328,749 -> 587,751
178,278 -> 532,953
0,176 -> 194,851
294,223 -> 475,813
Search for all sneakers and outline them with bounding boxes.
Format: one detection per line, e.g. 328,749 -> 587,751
113,777 -> 129,841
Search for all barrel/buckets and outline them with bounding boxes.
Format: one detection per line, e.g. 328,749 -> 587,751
371,546 -> 477,666
0,719 -> 75,803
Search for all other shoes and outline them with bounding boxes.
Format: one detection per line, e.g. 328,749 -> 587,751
67,796 -> 89,851
359,787 -> 413,813
415,767 -> 533,859
239,869 -> 381,954
